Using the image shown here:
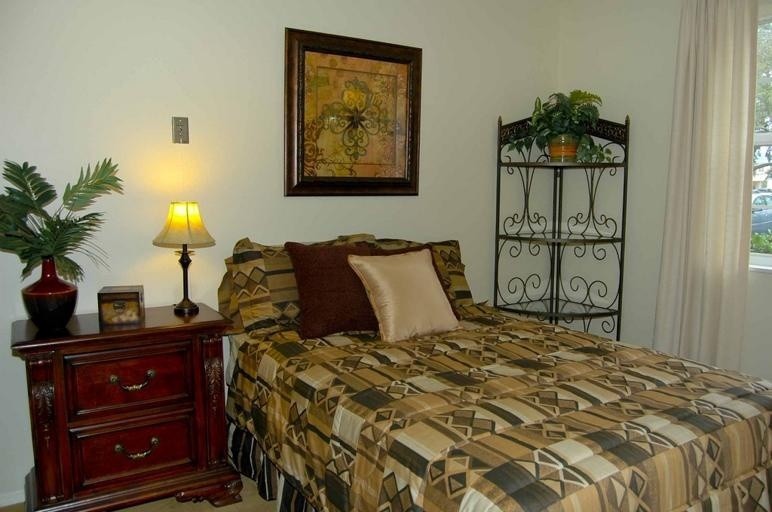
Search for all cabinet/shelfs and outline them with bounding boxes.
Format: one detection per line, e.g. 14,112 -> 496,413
494,112 -> 630,342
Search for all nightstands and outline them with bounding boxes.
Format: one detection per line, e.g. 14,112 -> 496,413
12,301 -> 245,511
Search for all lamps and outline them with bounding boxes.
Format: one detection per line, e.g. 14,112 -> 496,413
0,157 -> 125,330
153,202 -> 217,317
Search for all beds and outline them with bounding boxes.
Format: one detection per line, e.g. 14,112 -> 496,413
224,315 -> 772,512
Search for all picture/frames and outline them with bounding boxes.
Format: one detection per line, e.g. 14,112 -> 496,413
284,26 -> 422,197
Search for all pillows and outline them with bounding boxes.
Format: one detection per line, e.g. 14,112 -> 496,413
218,233 -> 478,344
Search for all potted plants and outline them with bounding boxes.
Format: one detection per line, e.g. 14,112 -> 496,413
508,90 -> 612,163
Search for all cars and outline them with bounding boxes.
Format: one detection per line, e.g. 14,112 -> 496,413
751,193 -> 772,236
